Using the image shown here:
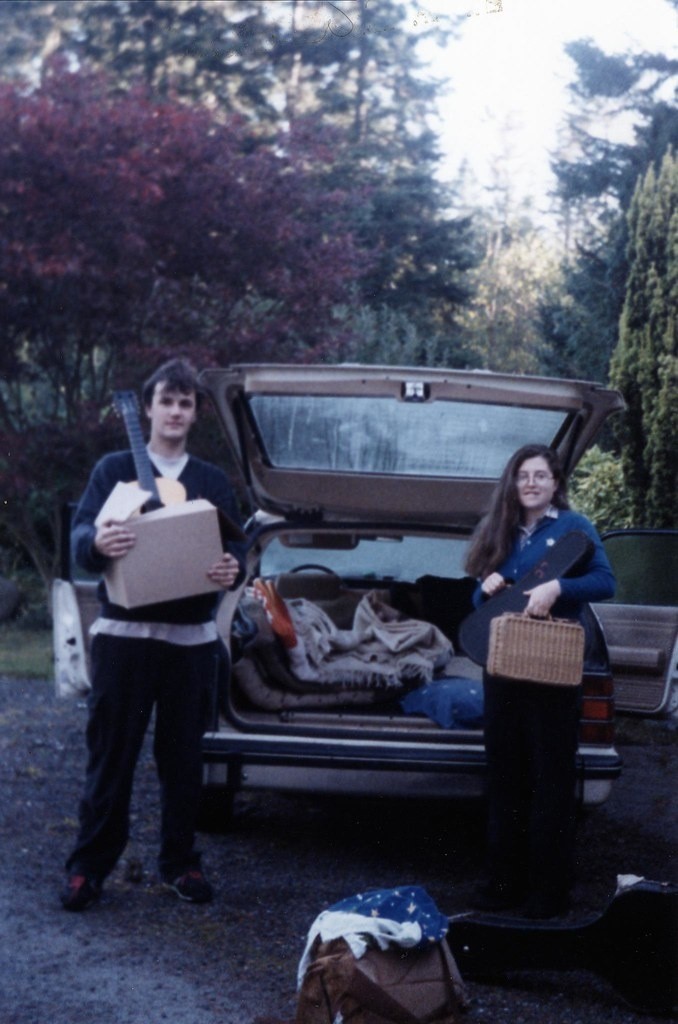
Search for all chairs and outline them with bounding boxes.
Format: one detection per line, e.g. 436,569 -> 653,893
274,574 -> 354,630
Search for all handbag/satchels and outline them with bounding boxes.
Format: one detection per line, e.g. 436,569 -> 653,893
297,932 -> 473,1024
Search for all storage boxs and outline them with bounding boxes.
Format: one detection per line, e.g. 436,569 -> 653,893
102,499 -> 228,608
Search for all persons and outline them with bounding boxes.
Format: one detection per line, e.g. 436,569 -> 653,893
63,358 -> 247,909
462,445 -> 617,919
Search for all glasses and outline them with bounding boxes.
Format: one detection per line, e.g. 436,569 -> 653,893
517,473 -> 554,484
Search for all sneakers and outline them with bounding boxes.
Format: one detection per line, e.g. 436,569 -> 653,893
60,875 -> 105,912
162,870 -> 215,902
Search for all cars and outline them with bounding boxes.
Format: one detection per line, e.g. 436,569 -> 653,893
173,360 -> 678,826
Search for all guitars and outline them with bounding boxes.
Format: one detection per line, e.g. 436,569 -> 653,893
111,389 -> 187,523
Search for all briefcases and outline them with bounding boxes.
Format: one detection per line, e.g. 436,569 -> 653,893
487,607 -> 584,685
